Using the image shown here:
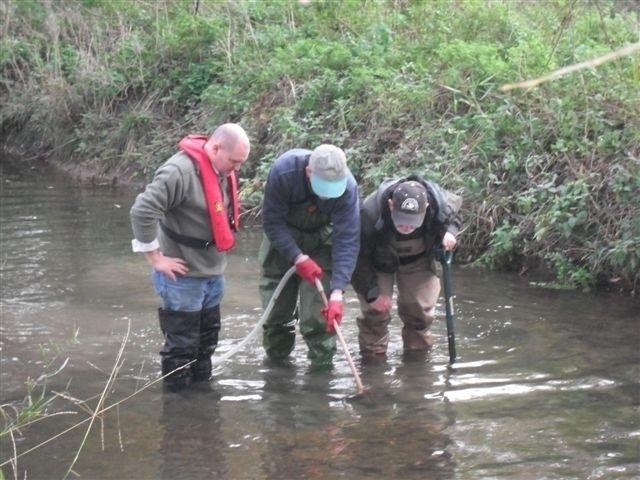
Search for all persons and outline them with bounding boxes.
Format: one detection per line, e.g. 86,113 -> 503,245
351,174 -> 463,365
258,144 -> 360,372
130,123 -> 250,393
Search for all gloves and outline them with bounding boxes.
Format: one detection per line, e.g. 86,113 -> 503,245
295,254 -> 322,286
321,293 -> 343,332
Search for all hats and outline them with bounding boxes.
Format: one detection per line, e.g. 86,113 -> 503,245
393,180 -> 427,226
310,144 -> 347,199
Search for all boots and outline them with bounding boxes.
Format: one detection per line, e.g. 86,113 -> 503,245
356,317 -> 434,355
262,307 -> 337,360
158,305 -> 221,385
